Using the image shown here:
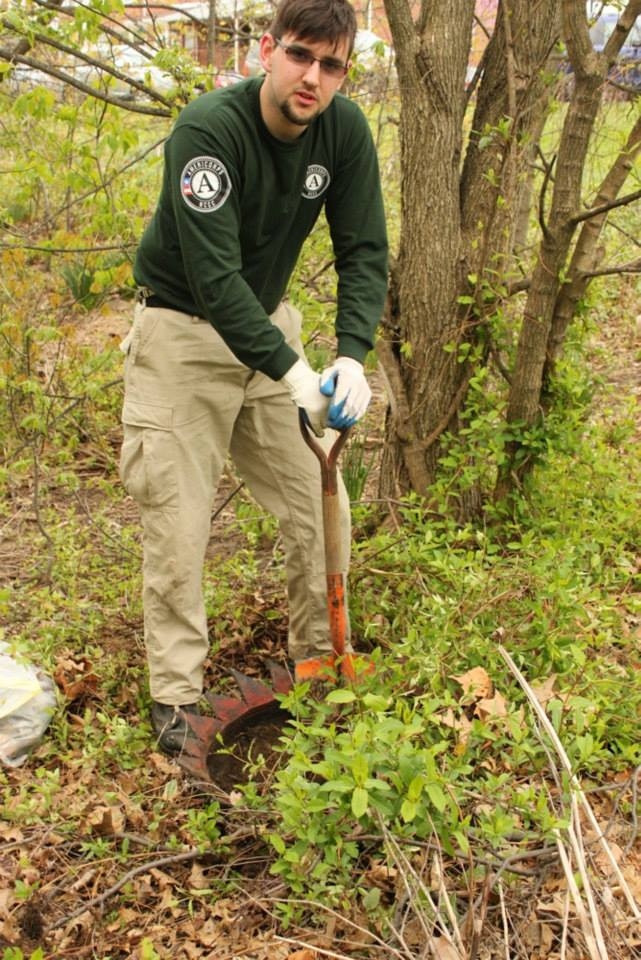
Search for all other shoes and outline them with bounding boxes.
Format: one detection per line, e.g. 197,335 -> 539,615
150,700 -> 201,757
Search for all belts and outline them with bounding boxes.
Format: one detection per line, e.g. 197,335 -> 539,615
138,292 -> 205,319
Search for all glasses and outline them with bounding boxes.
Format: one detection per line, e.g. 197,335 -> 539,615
275,36 -> 349,79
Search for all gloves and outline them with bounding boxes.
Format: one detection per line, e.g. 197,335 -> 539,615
283,358 -> 331,438
319,356 -> 371,431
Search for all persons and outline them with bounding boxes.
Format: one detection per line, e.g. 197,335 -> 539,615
118,1 -> 390,754
